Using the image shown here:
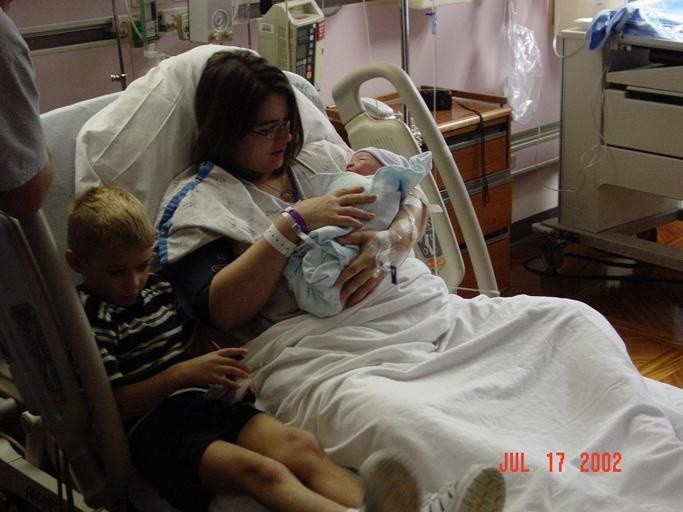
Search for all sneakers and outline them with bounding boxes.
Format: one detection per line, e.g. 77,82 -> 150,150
357,446 -> 420,511
419,462 -> 508,512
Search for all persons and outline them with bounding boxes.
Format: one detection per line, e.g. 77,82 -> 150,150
0,1 -> 58,222
66,179 -> 507,512
283,144 -> 411,318
154,44 -> 431,349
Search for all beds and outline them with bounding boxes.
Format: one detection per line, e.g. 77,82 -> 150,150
0,72 -> 683,511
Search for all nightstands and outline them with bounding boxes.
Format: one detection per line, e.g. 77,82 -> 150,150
323,83 -> 514,297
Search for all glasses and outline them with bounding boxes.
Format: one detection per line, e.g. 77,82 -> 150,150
249,120 -> 292,140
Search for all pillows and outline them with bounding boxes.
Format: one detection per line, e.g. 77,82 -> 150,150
71,40 -> 335,239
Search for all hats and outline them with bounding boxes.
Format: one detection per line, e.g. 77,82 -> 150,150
352,146 -> 410,168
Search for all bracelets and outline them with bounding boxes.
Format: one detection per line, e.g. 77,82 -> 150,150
263,224 -> 299,258
278,207 -> 307,237
